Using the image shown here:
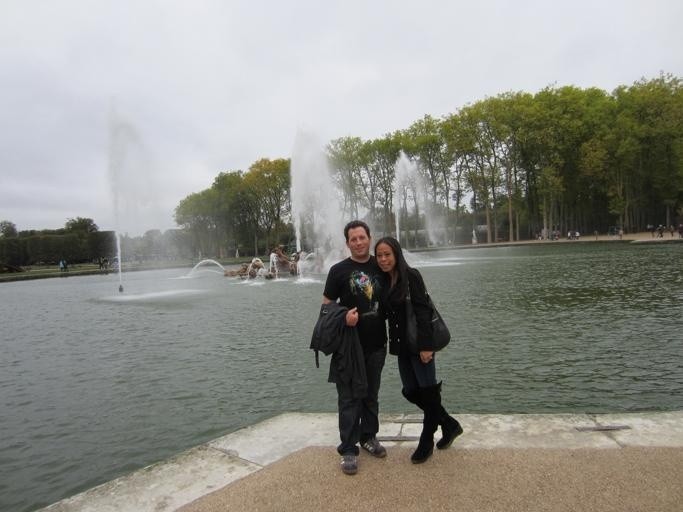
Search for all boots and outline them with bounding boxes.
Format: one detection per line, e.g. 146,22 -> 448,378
402,380 -> 463,464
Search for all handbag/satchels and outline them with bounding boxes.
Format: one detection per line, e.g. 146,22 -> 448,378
404,268 -> 451,352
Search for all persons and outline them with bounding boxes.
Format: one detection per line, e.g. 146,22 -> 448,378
375,236 -> 463,464
321,220 -> 388,475
535,228 -> 624,240
99,255 -> 110,272
59,255 -> 69,272
647,223 -> 683,239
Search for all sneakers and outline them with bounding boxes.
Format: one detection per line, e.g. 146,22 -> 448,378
341,455 -> 358,474
361,439 -> 387,458
